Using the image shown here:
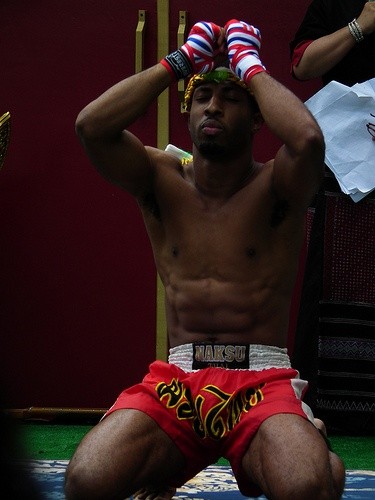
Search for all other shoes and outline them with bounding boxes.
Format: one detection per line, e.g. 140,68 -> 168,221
301,401 -> 325,429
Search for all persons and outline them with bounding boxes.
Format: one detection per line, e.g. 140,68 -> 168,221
59,19 -> 346,499
289,0 -> 374,439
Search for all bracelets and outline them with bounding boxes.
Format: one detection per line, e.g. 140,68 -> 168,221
348,19 -> 364,42
